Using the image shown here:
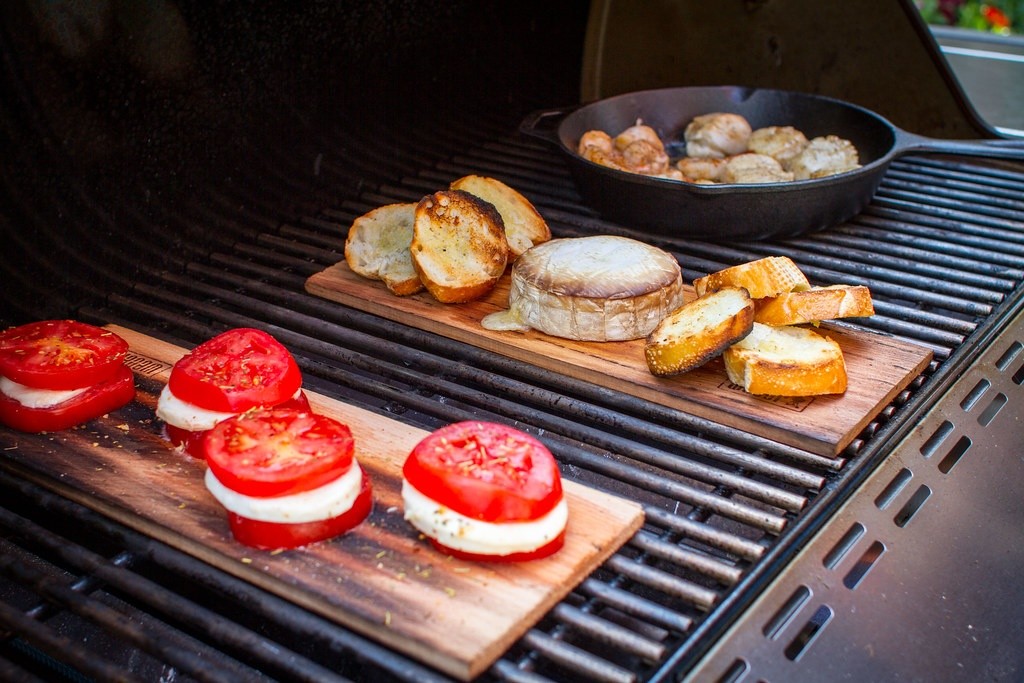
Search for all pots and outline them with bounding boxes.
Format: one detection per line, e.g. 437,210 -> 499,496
520,82 -> 1023,241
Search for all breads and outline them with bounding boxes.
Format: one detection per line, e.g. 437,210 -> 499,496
345,175 -> 874,396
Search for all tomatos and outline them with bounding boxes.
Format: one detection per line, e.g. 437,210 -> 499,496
0,319 -> 567,564
